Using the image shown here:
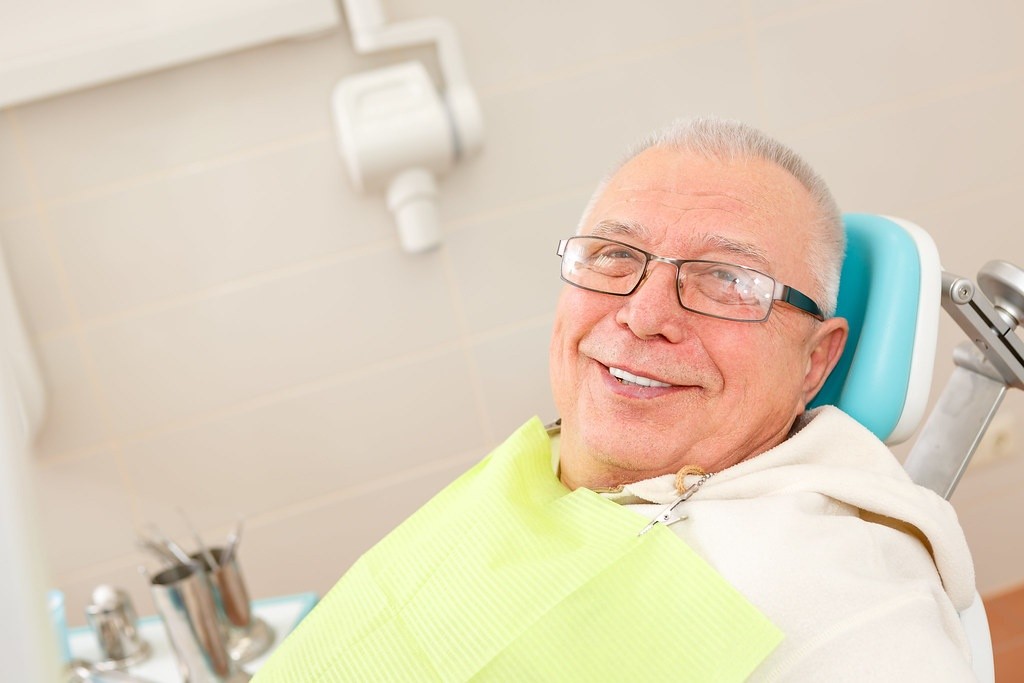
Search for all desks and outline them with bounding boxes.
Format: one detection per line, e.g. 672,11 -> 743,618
59,587 -> 322,683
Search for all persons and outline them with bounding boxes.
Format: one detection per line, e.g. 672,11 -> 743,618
246,117 -> 993,683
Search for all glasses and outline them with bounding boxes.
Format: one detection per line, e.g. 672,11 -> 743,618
556,235 -> 827,323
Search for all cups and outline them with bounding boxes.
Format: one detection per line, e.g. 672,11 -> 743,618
181,548 -> 250,640
150,561 -> 231,683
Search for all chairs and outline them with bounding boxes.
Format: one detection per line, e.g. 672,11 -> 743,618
807,216 -> 944,443
